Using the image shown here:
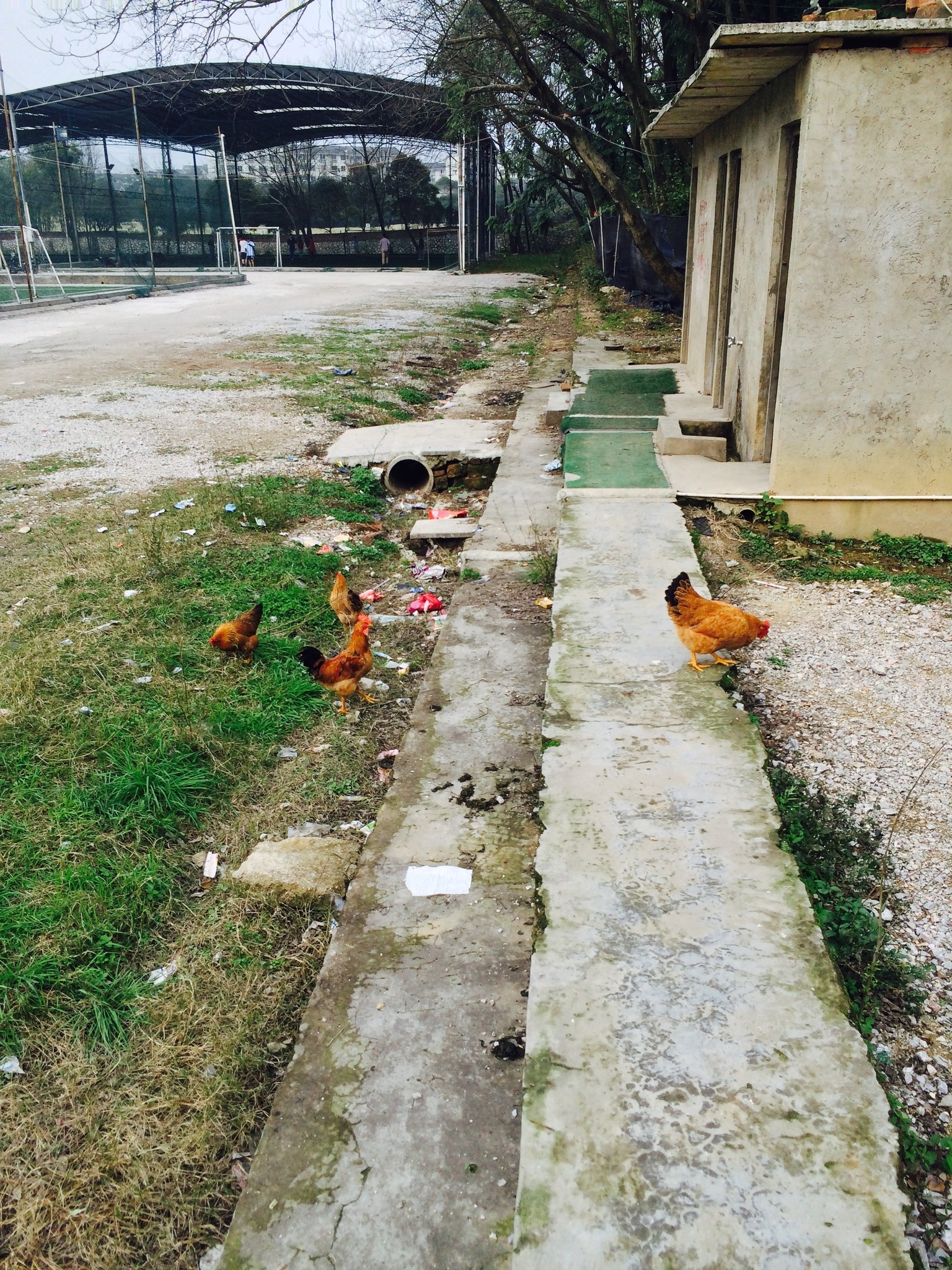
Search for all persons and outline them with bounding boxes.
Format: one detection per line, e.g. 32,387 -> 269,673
239,236 -> 248,267
379,233 -> 391,267
306,237 -> 317,262
233,235 -> 242,267
245,237 -> 255,267
287,234 -> 297,262
297,236 -> 304,258
353,235 -> 360,256
208,235 -> 216,264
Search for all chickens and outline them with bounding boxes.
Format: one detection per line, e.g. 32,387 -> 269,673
663,572 -> 771,673
209,572 -> 381,714
